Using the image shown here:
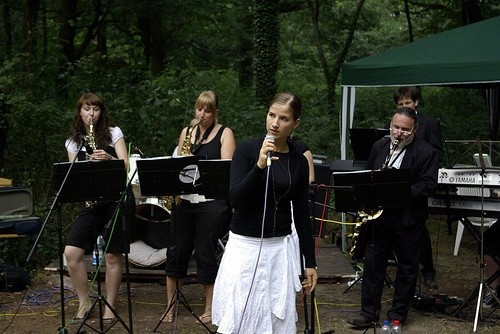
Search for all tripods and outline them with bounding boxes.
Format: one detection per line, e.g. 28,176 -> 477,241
136,156 -> 214,334
53,159 -> 134,334
444,135 -> 500,334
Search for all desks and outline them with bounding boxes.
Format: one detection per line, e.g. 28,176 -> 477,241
0,234 -> 30,237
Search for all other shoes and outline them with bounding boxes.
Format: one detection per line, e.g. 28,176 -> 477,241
389,319 -> 405,328
425,279 -> 437,289
346,315 -> 379,326
483,285 -> 500,309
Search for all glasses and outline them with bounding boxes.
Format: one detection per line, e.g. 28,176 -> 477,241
389,118 -> 415,135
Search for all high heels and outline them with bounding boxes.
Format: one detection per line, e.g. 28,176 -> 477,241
70,309 -> 92,325
102,317 -> 114,323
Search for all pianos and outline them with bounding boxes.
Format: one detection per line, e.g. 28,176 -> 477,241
428,163 -> 500,317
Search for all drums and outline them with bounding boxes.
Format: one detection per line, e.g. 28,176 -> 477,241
120,197 -> 172,268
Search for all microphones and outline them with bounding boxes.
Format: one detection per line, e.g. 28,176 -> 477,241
263,136 -> 275,167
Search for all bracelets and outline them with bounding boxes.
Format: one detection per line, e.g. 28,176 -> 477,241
106,152 -> 112,160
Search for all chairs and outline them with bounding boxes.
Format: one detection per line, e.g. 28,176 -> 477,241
452,217 -> 496,258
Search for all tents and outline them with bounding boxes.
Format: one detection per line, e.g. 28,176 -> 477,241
342,15 -> 500,254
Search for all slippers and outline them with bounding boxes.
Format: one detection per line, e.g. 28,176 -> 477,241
160,313 -> 175,323
195,315 -> 211,323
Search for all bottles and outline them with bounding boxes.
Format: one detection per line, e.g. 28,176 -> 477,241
391,320 -> 402,334
92,235 -> 106,266
380,321 -> 391,334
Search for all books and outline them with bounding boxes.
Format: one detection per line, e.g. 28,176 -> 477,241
473,153 -> 492,167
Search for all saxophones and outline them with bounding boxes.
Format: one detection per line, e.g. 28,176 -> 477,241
85,117 -> 99,209
349,138 -> 402,257
159,118 -> 202,211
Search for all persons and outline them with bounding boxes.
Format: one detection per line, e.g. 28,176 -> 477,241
482,284 -> 500,309
159,90 -> 236,325
347,106 -> 439,330
212,92 -> 319,334
64,93 -> 130,324
390,87 -> 445,288
